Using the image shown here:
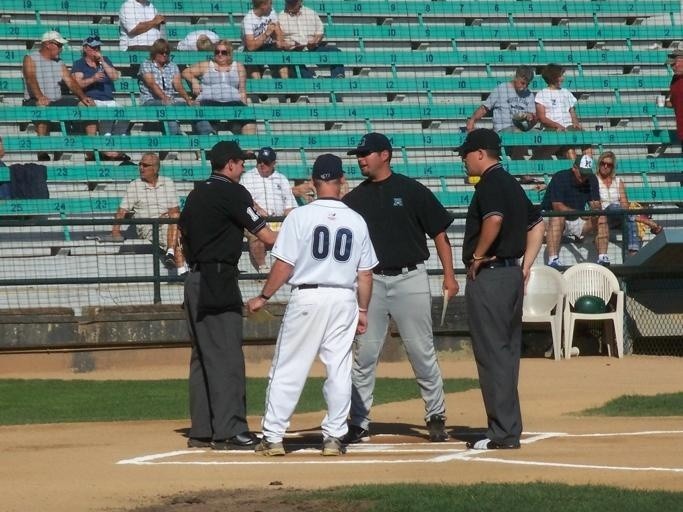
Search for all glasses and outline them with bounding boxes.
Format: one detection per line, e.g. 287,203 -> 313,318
50,41 -> 64,49
215,49 -> 226,55
84,36 -> 100,43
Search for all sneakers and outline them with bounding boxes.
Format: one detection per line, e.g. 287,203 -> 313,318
548,258 -> 563,266
465,438 -> 521,449
427,414 -> 450,442
165,251 -> 177,266
344,427 -> 369,442
322,438 -> 346,455
187,432 -> 287,456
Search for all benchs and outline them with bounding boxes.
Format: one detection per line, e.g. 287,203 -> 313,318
0,0 -> 683,256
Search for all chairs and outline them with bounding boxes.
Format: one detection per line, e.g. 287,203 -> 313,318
516,263 -> 567,361
562,261 -> 625,360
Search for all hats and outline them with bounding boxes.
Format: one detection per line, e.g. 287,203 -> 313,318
666,50 -> 682,65
82,39 -> 103,47
210,141 -> 248,165
452,128 -> 501,152
312,154 -> 346,179
346,133 -> 391,155
575,153 -> 594,176
258,148 -> 277,164
42,31 -> 68,45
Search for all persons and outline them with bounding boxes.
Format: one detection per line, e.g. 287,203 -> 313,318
181,39 -> 258,160
112,153 -> 193,279
180,142 -> 305,450
22,30 -> 97,163
177,30 -> 220,69
136,38 -> 217,136
668,37 -> 683,147
536,64 -> 593,161
278,1 -> 345,79
119,0 -> 167,77
242,1 -> 292,102
451,129 -> 544,450
314,132 -> 460,443
536,155 -> 609,274
467,66 -> 547,186
229,147 -> 304,275
589,152 -> 644,264
246,154 -> 371,456
291,173 -> 350,205
71,35 -> 131,163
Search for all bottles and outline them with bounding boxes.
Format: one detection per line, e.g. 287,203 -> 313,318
97,61 -> 104,82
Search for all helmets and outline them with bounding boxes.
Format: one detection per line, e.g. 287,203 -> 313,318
576,296 -> 606,315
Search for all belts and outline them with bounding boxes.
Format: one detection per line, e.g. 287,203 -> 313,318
298,284 -> 317,289
478,259 -> 516,268
374,266 -> 416,275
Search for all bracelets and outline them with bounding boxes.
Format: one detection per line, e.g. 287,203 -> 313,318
359,308 -> 368,312
260,293 -> 270,300
472,253 -> 485,261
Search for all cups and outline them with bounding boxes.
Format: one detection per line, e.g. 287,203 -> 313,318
657,95 -> 666,108
595,124 -> 603,130
